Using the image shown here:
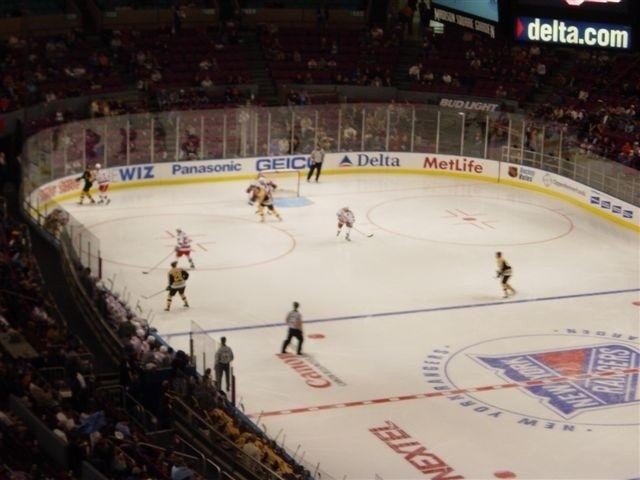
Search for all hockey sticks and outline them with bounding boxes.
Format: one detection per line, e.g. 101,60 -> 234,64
350,225 -> 375,238
141,288 -> 167,300
142,250 -> 175,275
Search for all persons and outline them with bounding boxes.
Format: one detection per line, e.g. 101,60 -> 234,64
1,0 -> 640,171
307,142 -> 325,182
336,204 -> 355,242
1,166 -> 306,479
496,252 -> 516,298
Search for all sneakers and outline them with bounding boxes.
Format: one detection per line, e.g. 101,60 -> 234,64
76,199 -> 110,206
502,290 -> 516,298
336,231 -> 352,242
164,265 -> 196,311
258,215 -> 284,223
306,178 -> 320,183
282,350 -> 303,355
